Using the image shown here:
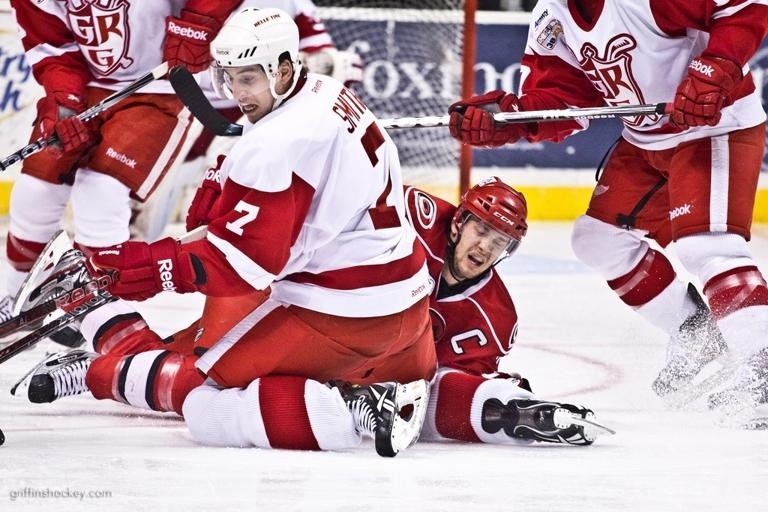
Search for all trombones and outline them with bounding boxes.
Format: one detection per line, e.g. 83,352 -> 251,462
169,65 -> 673,137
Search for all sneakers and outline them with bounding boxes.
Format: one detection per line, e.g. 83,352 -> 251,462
654,285 -> 727,394
27,348 -> 99,402
0,250 -> 92,347
709,348 -> 768,410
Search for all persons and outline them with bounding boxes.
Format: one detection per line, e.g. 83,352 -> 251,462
14,176 -> 532,417
448,0 -> 766,429
91,5 -> 618,457
139,1 -> 365,247
0,2 -> 238,352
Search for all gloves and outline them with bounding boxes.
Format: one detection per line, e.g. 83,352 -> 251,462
37,94 -> 98,157
84,236 -> 197,299
449,90 -> 538,148
671,57 -> 741,127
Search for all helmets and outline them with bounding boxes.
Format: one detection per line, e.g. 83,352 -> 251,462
452,178 -> 528,257
206,5 -> 303,106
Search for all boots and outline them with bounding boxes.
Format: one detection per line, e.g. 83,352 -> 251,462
328,375 -> 397,455
482,398 -> 595,447
